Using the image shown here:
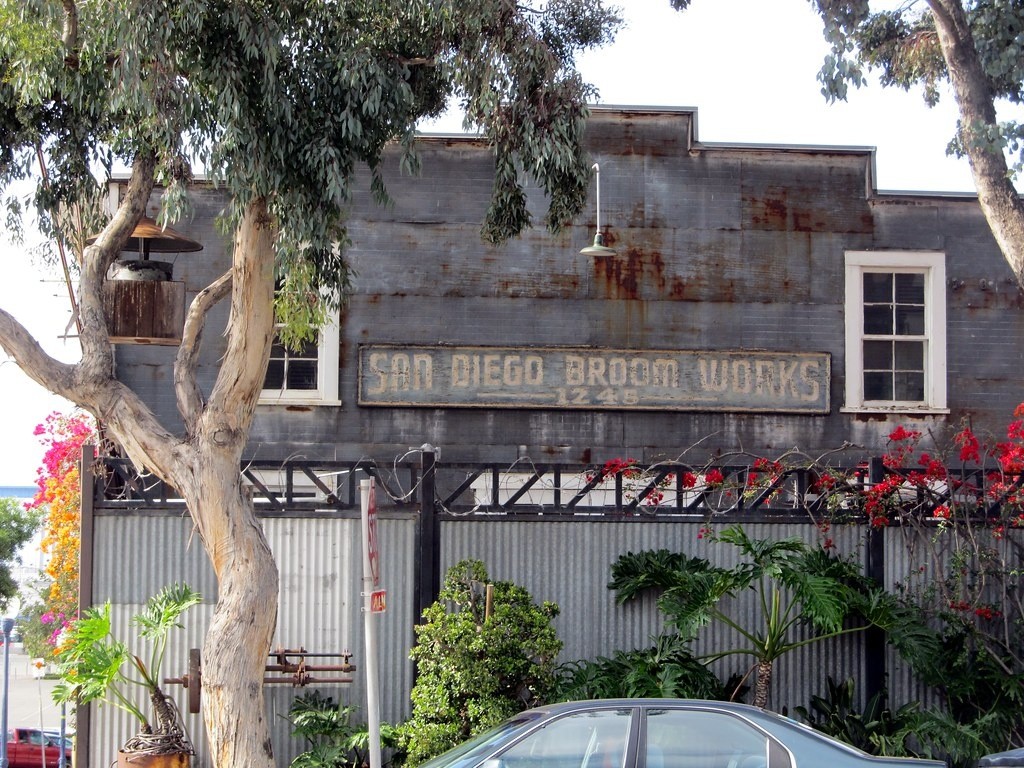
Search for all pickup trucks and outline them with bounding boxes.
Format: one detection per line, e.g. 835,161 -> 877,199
6,728 -> 72,768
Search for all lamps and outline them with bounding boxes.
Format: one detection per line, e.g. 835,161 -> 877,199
579,162 -> 619,259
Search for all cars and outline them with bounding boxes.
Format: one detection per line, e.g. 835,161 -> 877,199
417,697 -> 947,768
42,733 -> 73,748
10,626 -> 24,636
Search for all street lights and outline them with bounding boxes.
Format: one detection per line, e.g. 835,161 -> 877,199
0,594 -> 20,768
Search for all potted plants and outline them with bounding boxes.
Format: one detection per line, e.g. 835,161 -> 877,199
53,580 -> 202,768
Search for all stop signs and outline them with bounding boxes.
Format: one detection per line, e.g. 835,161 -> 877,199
366,476 -> 379,587
